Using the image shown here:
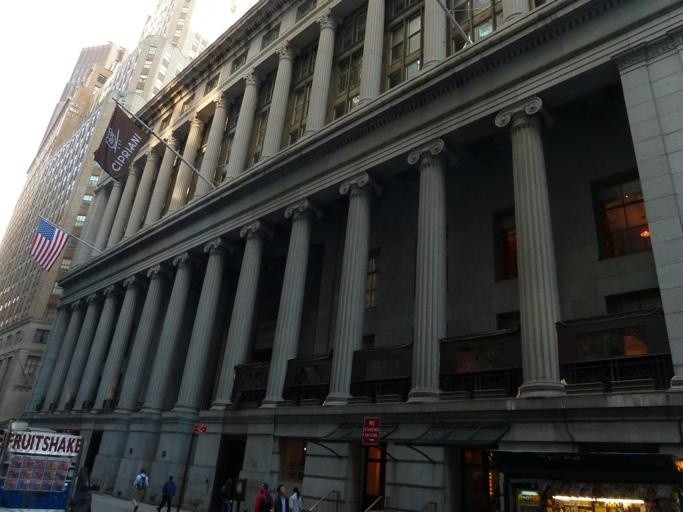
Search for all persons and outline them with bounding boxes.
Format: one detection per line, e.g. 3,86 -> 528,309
157,474 -> 177,512
220,478 -> 233,512
255,482 -> 273,512
288,486 -> 304,512
273,483 -> 289,512
130,468 -> 149,512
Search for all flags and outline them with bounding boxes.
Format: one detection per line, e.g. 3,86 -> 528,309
93,104 -> 150,182
29,219 -> 70,271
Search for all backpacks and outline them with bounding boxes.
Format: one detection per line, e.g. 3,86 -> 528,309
135,474 -> 147,490
